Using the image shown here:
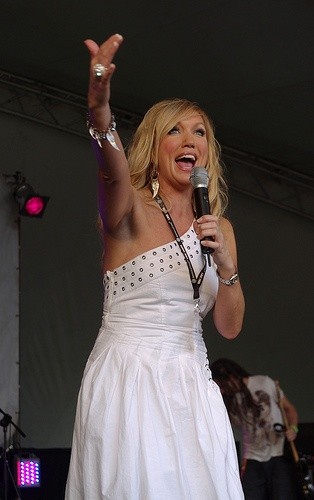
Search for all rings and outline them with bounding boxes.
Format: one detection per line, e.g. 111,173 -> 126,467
94,64 -> 108,78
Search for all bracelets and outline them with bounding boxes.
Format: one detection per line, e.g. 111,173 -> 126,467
289,425 -> 298,433
85,112 -> 120,151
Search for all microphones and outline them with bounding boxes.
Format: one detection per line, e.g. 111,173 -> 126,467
190,166 -> 215,254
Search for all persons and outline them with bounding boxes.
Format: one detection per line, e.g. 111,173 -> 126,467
209,358 -> 298,500
64,32 -> 245,500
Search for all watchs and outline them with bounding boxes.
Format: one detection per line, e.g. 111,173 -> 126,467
216,269 -> 239,286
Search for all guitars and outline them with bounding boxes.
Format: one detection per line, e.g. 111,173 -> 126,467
275,380 -> 313,500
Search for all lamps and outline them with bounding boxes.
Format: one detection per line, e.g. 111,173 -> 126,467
0,410 -> 41,500
4,171 -> 51,219
